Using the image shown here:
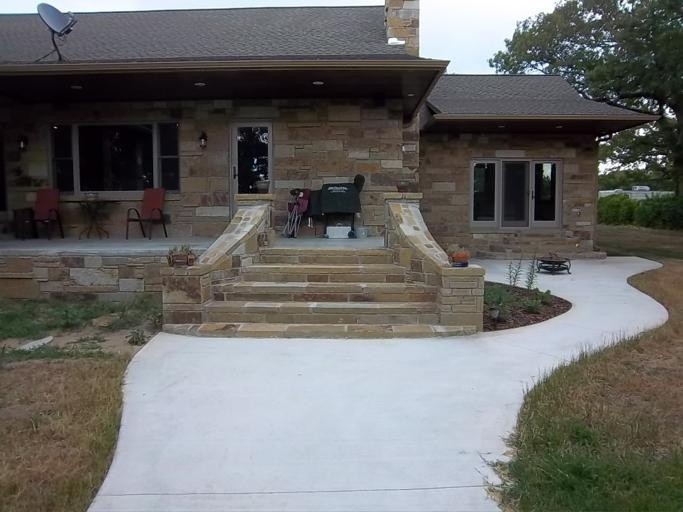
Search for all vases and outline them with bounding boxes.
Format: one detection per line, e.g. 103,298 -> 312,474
452,261 -> 468,267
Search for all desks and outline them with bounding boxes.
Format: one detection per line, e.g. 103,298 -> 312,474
77,200 -> 120,240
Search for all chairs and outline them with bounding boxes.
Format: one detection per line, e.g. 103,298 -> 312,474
22,189 -> 65,240
126,187 -> 169,241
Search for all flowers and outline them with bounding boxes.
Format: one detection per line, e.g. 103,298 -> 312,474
445,244 -> 470,262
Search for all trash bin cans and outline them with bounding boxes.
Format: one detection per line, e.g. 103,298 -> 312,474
14,208 -> 35,238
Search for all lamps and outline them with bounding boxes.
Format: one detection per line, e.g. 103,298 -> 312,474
198,131 -> 208,149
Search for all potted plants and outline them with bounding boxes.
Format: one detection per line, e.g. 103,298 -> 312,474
168,240 -> 192,266
